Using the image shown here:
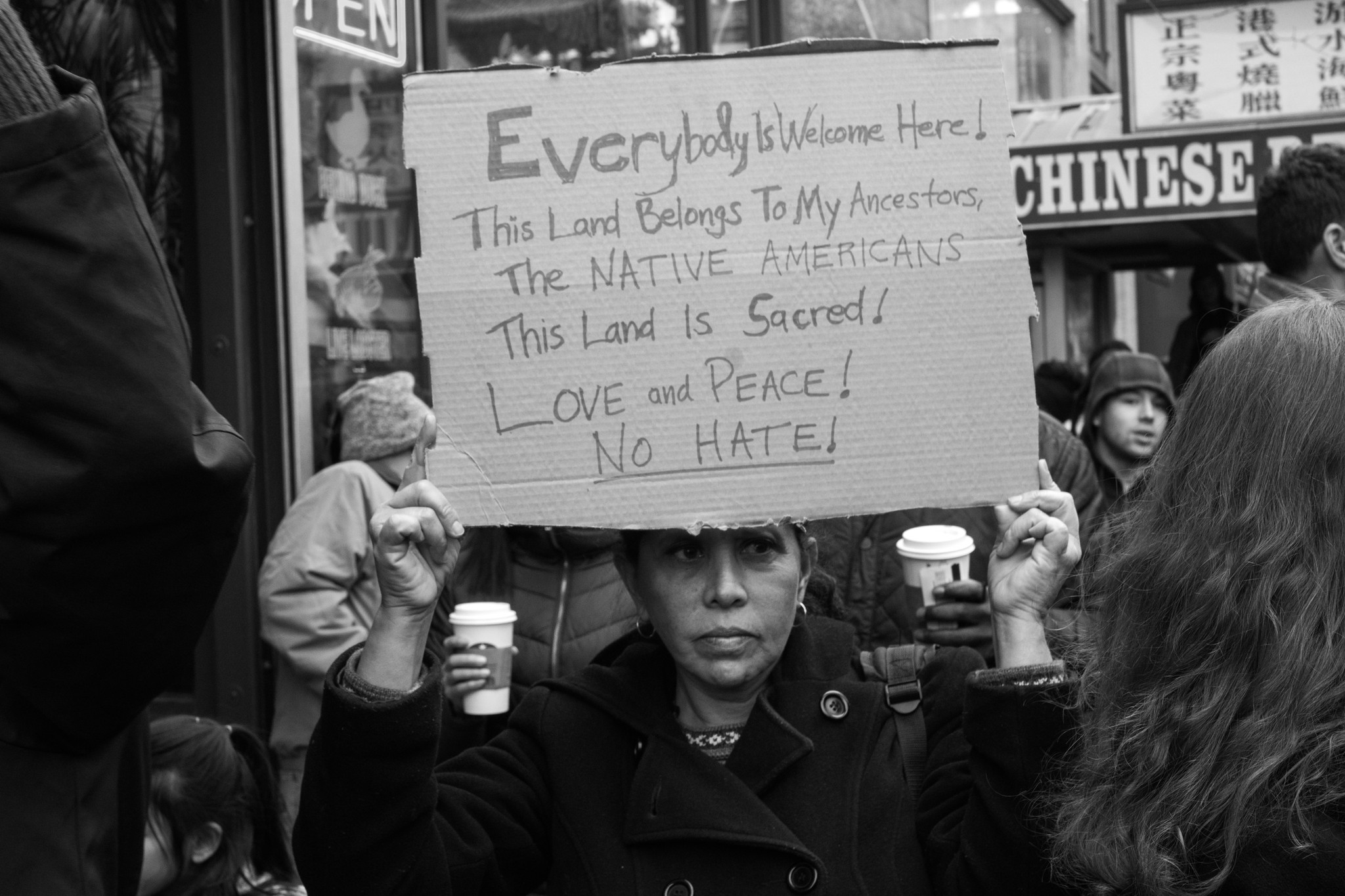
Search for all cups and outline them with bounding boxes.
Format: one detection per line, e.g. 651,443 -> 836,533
449,601 -> 518,716
896,525 -> 975,631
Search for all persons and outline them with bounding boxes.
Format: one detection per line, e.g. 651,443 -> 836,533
139,716 -> 307,896
423,144 -> 1345,689
0,0 -> 255,896
292,443 -> 1075,896
1032,296 -> 1345,896
259,372 -> 436,835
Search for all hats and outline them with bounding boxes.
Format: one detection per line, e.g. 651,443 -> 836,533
340,370 -> 438,461
